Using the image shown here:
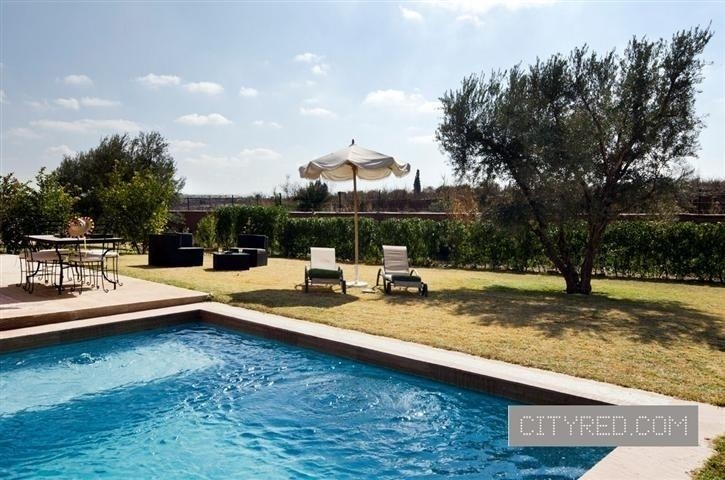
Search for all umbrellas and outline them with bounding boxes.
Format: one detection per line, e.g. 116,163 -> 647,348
298,138 -> 411,283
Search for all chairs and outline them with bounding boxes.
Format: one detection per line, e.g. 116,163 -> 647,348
377,245 -> 427,297
304,247 -> 346,294
16,219 -> 124,295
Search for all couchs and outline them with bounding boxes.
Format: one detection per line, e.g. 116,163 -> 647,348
148,232 -> 269,269
